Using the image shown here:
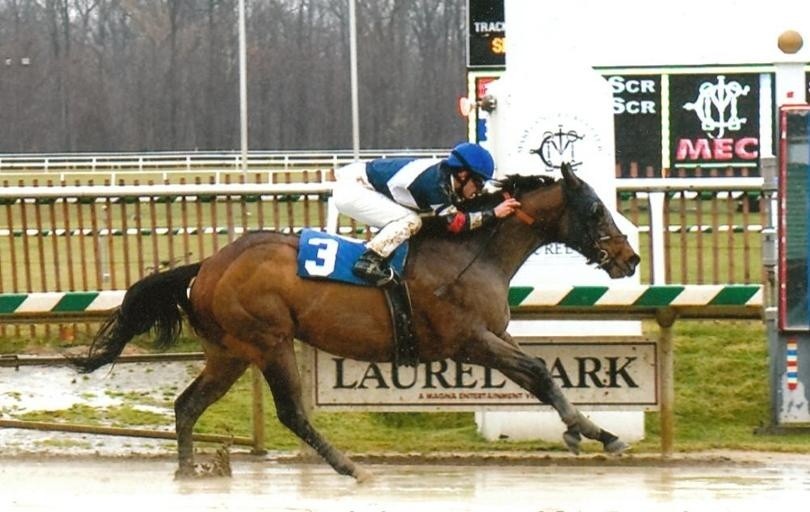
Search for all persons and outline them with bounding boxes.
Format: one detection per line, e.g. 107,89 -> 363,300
332,141 -> 523,290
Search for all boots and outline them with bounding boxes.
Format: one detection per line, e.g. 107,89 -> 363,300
354,248 -> 399,287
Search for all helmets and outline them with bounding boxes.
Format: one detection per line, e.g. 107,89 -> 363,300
443,143 -> 495,181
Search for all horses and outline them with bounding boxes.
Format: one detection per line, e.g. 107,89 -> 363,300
56,161 -> 642,489
